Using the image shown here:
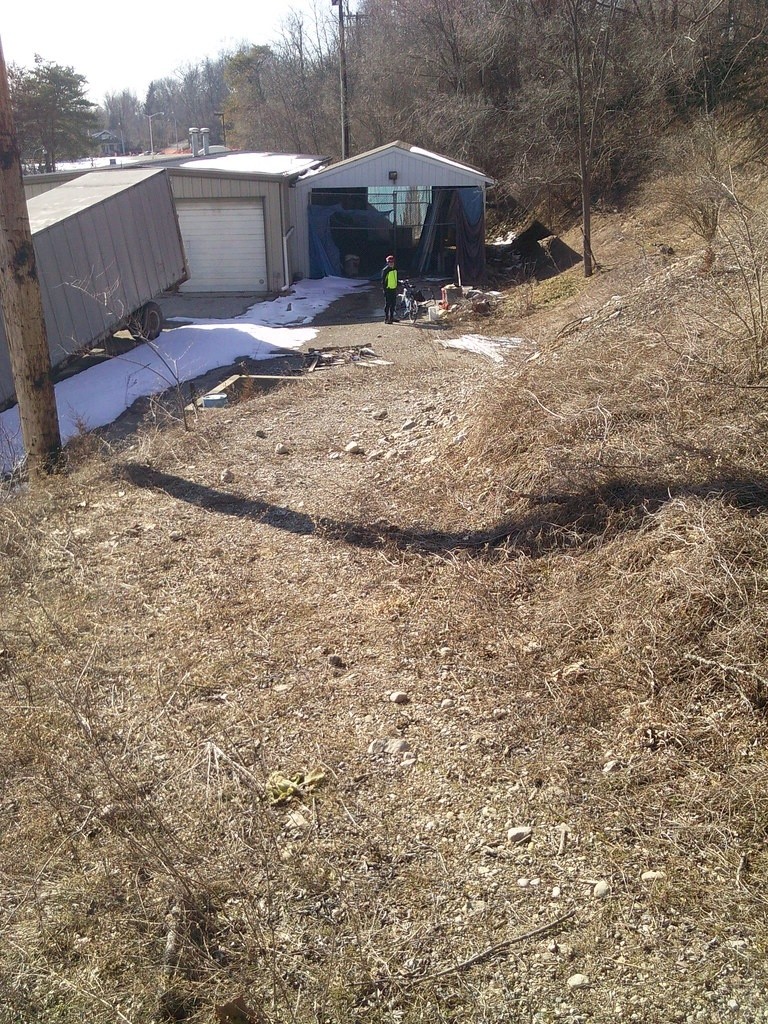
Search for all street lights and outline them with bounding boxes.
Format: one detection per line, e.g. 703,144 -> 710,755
139,111 -> 164,160
118,117 -> 126,155
32,145 -> 48,174
213,112 -> 226,147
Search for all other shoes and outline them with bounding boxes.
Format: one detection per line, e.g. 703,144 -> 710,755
390,317 -> 399,322
385,320 -> 392,324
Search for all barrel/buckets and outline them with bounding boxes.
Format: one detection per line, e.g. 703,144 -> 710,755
203,393 -> 227,408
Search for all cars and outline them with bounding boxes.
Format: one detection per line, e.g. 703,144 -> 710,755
139,151 -> 152,156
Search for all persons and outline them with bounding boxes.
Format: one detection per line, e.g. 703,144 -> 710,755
381,256 -> 399,324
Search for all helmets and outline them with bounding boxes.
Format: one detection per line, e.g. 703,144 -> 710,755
385,256 -> 394,263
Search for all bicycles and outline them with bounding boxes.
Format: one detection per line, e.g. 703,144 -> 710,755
395,277 -> 419,324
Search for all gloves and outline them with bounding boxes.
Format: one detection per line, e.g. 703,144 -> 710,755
384,294 -> 387,297
